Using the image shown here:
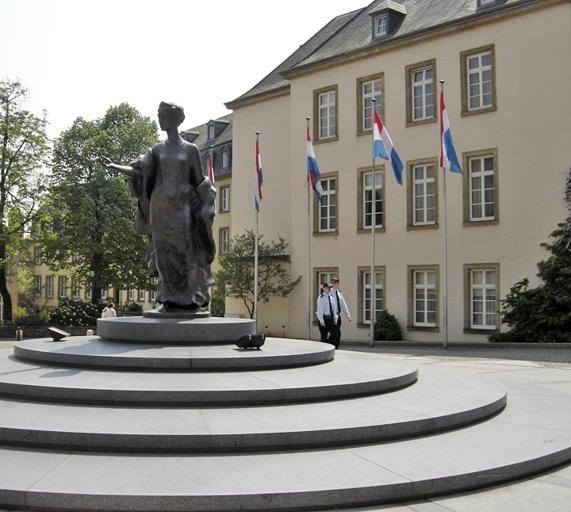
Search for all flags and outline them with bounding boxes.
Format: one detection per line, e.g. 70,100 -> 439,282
205,144 -> 217,191
253,131 -> 264,214
370,95 -> 405,191
305,116 -> 326,208
440,79 -> 466,178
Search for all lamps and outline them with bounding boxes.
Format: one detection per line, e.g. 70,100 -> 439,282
47,326 -> 70,342
237,333 -> 267,352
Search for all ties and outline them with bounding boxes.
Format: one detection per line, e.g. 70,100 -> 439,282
328,296 -> 334,316
336,291 -> 341,313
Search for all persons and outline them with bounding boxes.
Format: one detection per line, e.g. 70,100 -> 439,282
331,278 -> 353,349
99,99 -> 221,313
317,283 -> 337,342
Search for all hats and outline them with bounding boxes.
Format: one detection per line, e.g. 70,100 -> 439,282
330,278 -> 339,283
323,283 -> 333,288
